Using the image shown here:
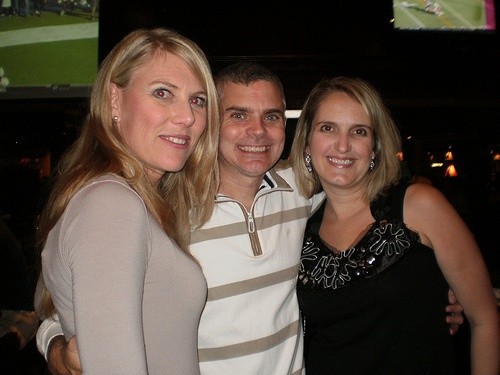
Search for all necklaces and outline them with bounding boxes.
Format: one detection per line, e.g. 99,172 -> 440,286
37,62 -> 466,375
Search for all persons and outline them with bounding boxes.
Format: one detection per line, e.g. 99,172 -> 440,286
42,27 -> 223,375
290,75 -> 499,375
